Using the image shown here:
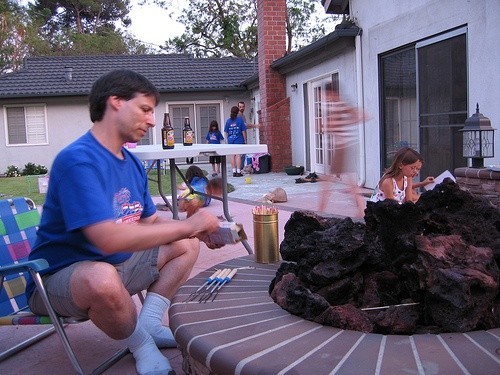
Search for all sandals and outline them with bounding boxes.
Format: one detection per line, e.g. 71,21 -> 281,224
305,172 -> 319,179
295,178 -> 305,183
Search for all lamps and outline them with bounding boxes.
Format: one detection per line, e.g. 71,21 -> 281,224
458,102 -> 497,168
251,97 -> 255,102
290,83 -> 298,92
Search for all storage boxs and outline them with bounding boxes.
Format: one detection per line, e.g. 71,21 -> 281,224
39,178 -> 50,193
209,221 -> 248,245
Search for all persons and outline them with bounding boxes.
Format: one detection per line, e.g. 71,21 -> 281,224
317,80 -> 369,218
27,69 -> 226,375
369,146 -> 435,205
205,101 -> 262,177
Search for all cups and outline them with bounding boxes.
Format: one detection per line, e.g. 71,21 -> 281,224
253,211 -> 279,264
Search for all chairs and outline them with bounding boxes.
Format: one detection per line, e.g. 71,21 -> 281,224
0,198 -> 146,375
144,158 -> 167,175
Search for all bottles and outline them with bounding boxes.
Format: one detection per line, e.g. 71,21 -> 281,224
161,113 -> 174,149
183,117 -> 193,146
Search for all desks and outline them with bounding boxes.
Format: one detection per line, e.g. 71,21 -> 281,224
123,142 -> 268,254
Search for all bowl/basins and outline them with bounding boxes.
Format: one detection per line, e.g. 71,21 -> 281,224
283,166 -> 304,176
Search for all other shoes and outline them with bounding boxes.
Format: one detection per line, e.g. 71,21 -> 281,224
233,173 -> 243,177
